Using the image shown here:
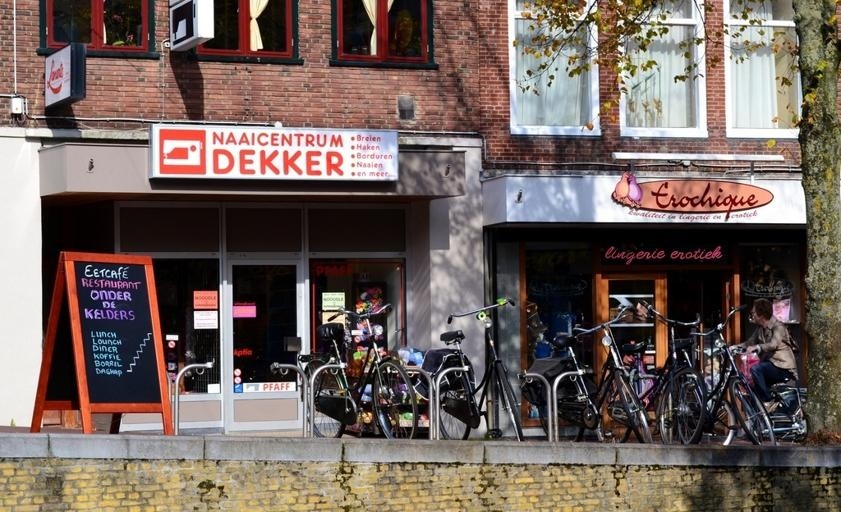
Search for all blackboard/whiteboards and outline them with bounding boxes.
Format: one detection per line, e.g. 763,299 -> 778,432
38,251 -> 77,411
64,252 -> 170,412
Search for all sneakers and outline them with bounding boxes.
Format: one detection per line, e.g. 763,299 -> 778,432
760,400 -> 779,416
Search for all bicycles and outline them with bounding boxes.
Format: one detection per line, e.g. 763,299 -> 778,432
429,294 -> 527,441
298,299 -> 420,441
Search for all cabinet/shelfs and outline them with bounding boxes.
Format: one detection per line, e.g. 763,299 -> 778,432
593,266 -> 669,440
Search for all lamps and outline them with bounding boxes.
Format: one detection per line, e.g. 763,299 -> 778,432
513,188 -> 524,209
440,163 -> 453,180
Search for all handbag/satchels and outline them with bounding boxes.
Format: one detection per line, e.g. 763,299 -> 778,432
521,357 -> 597,403
414,348 -> 475,400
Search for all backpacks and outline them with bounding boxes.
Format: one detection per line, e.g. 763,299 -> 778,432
772,323 -> 799,352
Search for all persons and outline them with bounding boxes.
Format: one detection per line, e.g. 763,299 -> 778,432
727,296 -> 801,438
703,357 -> 722,410
599,342 -> 656,443
